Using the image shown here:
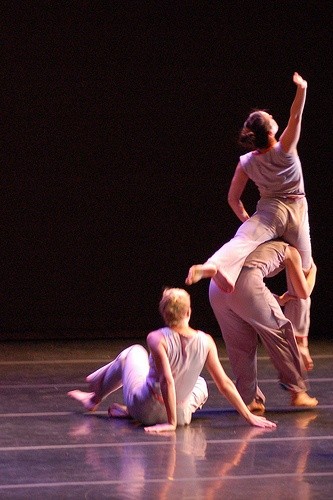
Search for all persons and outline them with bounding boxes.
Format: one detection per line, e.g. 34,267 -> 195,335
185,73 -> 313,369
208,240 -> 318,412
66,287 -> 276,432
